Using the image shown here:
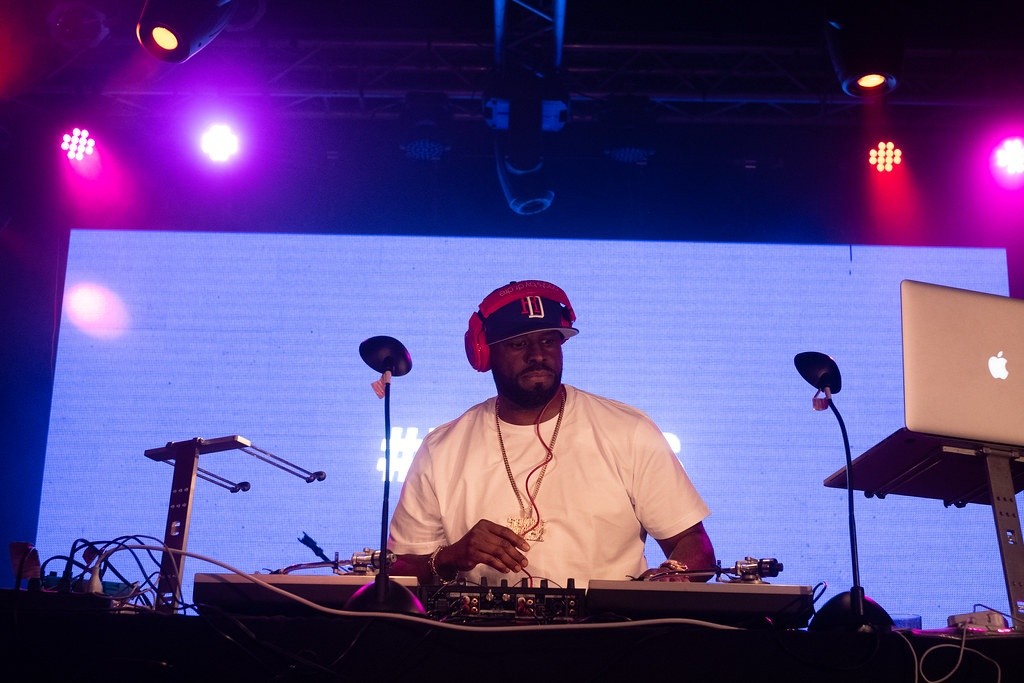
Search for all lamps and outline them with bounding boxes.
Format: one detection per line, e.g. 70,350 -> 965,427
833,16 -> 898,100
134,0 -> 233,65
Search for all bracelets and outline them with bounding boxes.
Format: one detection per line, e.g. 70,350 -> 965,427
660,560 -> 691,578
429,546 -> 459,582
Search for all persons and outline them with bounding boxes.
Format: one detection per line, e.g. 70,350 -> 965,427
381,280 -> 716,582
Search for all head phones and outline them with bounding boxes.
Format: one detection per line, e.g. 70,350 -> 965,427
464,280 -> 576,372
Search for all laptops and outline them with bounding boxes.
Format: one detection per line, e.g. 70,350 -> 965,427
823,279 -> 1024,505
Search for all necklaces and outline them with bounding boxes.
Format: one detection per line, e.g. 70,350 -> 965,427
495,389 -> 566,541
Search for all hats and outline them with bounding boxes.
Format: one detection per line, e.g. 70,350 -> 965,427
486,281 -> 580,345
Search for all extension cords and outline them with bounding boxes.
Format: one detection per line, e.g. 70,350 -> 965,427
946,609 -> 1006,628
